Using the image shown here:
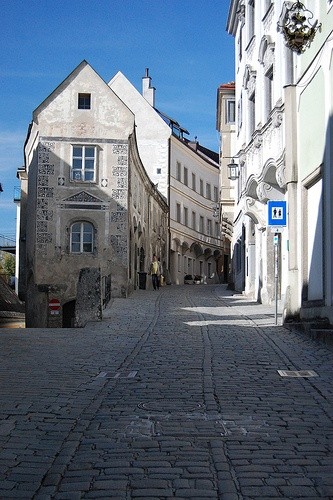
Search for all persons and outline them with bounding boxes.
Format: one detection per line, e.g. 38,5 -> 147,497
148,255 -> 163,291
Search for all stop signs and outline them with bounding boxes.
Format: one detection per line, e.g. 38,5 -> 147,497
49,299 -> 61,311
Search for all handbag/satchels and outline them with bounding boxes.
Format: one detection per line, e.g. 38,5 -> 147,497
160,275 -> 164,286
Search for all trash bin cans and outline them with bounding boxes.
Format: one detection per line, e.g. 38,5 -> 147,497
138,271 -> 147,290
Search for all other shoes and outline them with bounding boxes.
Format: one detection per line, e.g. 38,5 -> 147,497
157,287 -> 159,290
153,289 -> 156,291
